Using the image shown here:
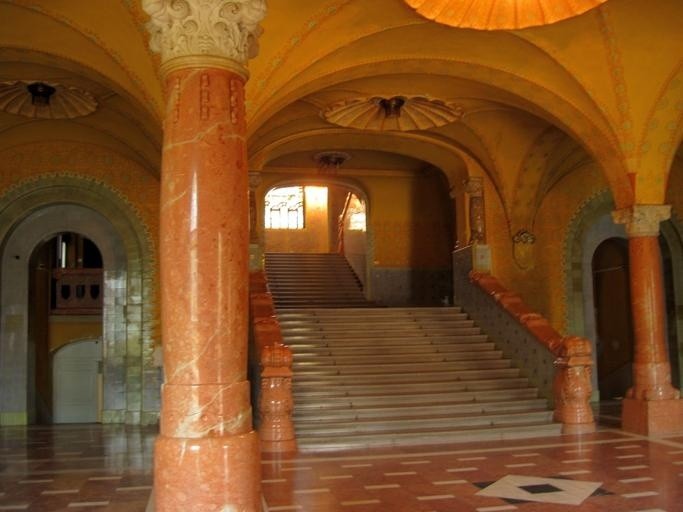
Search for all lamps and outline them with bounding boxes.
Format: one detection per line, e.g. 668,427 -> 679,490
0,78 -> 99,122
314,150 -> 351,170
321,92 -> 465,131
